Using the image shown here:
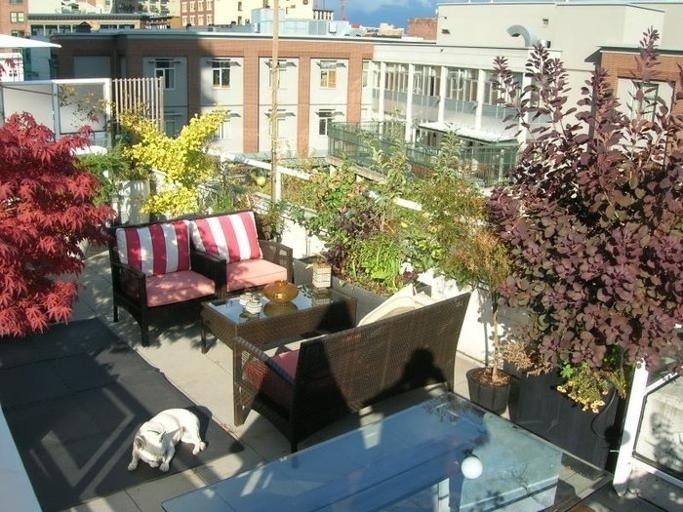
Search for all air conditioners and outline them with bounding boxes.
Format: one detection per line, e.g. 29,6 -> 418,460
125,408 -> 207,474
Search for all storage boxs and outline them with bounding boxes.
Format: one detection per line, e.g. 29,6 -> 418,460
230,291 -> 472,460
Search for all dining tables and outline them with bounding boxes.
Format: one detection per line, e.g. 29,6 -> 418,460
448,217 -> 515,416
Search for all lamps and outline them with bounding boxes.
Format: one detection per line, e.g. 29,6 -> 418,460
186,211 -> 293,303
611,322 -> 682,512
105,220 -> 224,347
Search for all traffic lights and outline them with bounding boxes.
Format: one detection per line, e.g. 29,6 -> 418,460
114,221 -> 191,275
186,211 -> 263,263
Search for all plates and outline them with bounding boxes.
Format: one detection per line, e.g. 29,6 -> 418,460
160,391 -> 613,512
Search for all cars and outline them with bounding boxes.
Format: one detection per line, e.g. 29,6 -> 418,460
239,294 -> 255,309
245,301 -> 263,314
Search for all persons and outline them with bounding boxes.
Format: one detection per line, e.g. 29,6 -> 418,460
164,20 -> 247,31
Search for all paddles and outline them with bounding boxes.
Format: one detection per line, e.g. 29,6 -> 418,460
0,311 -> 242,512
